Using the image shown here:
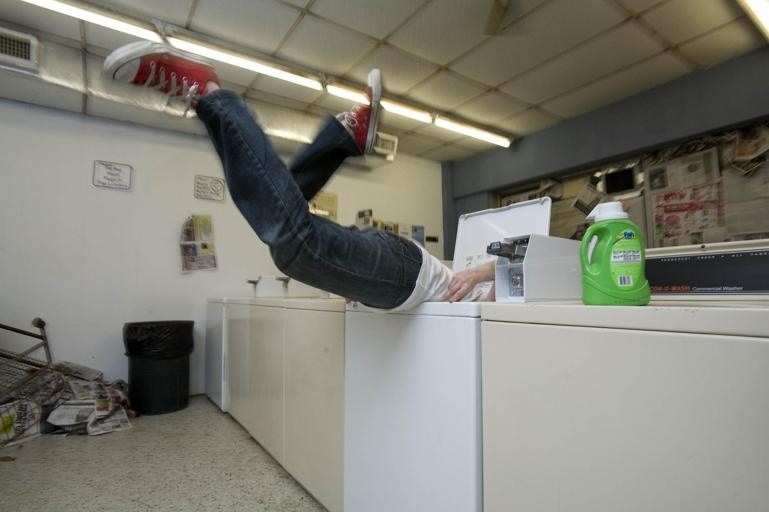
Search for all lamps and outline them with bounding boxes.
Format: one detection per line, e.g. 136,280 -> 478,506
166,35 -> 324,92
16,0 -> 166,43
327,83 -> 433,125
435,114 -> 511,149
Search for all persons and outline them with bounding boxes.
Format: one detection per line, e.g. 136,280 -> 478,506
99,40 -> 496,312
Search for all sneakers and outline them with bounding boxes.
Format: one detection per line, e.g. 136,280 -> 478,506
102,39 -> 222,110
340,67 -> 384,155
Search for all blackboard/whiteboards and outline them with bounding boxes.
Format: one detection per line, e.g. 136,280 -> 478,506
451,196 -> 552,299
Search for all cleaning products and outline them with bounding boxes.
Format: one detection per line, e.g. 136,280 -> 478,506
578,202 -> 652,305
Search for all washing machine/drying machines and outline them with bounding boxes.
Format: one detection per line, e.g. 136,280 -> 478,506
340,197 -> 554,511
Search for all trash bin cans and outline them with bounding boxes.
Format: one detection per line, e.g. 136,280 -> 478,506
122,321 -> 195,416
